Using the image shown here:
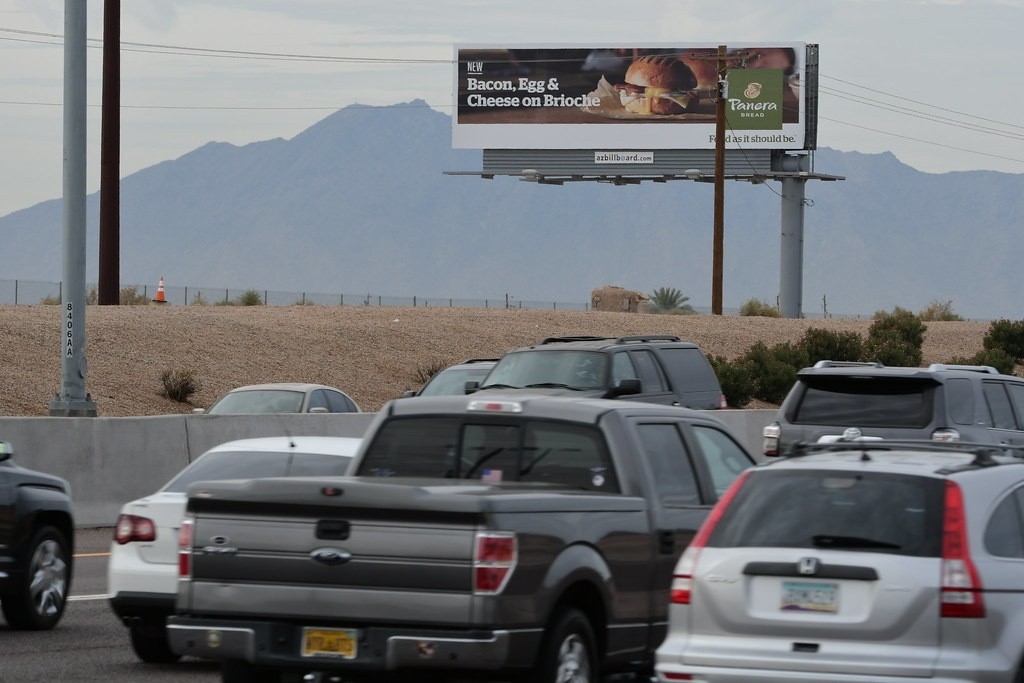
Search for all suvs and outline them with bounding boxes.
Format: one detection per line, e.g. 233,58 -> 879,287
402,355 -> 502,399
762,358 -> 1023,458
647,434 -> 1023,683
462,331 -> 731,412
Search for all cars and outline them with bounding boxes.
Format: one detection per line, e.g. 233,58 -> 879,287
103,435 -> 476,667
0,438 -> 80,630
191,381 -> 363,414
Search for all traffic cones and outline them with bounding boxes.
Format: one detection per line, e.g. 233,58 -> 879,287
151,275 -> 168,303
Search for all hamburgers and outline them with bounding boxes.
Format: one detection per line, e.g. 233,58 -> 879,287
613,54 -> 700,114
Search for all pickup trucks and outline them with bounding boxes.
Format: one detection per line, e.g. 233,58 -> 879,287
163,391 -> 761,683
